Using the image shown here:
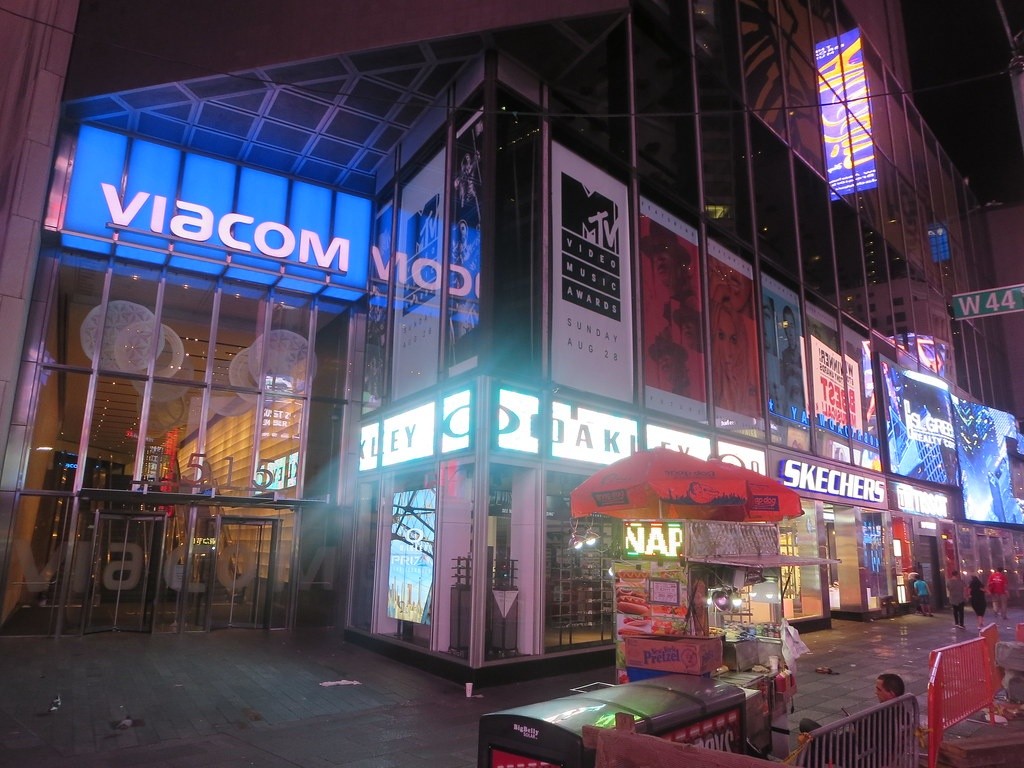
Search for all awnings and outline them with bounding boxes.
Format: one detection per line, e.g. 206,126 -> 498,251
688,552 -> 844,602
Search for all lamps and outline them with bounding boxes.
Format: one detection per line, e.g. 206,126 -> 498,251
570,517 -> 601,547
690,564 -> 734,612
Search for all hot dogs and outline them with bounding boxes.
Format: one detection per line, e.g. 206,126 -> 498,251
613,571 -> 653,635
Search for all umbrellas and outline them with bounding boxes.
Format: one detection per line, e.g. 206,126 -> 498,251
570,444 -> 805,526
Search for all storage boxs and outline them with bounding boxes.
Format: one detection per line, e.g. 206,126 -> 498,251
624,634 -> 723,674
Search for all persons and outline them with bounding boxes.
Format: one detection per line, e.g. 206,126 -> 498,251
710,301 -> 760,419
690,580 -> 708,636
762,294 -> 778,357
913,574 -> 934,616
673,296 -> 701,352
653,245 -> 706,404
450,149 -> 482,341
988,567 -> 1009,620
947,571 -> 966,629
797,674 -> 911,768
778,306 -> 807,421
970,570 -> 987,630
650,334 -> 691,399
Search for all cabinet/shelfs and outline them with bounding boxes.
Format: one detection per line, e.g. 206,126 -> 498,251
547,532 -> 613,630
